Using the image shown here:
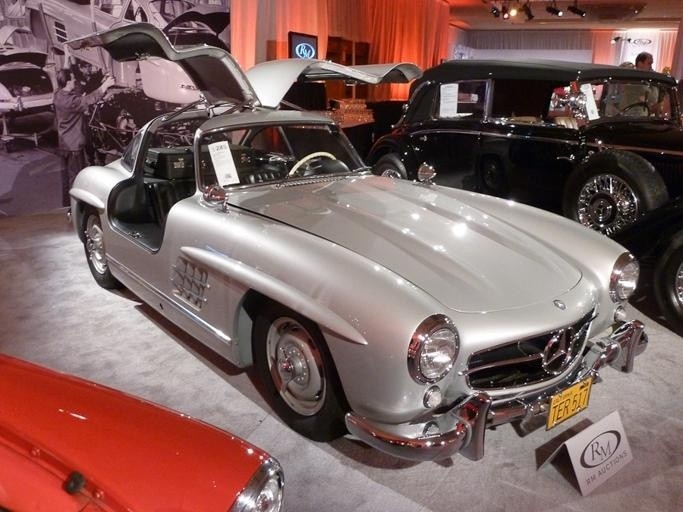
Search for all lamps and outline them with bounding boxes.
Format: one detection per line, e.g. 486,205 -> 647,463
481,0 -> 586,21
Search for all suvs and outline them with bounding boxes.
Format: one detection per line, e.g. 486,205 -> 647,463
366,56 -> 682,333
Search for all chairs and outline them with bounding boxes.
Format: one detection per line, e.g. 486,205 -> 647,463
146,148 -> 279,231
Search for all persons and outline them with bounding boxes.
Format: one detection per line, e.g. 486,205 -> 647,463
66,46 -> 108,165
52,44 -> 116,207
548,52 -> 666,128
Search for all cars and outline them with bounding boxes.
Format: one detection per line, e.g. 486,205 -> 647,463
2,353 -> 284,512
0,16 -> 200,146
63,21 -> 649,462
